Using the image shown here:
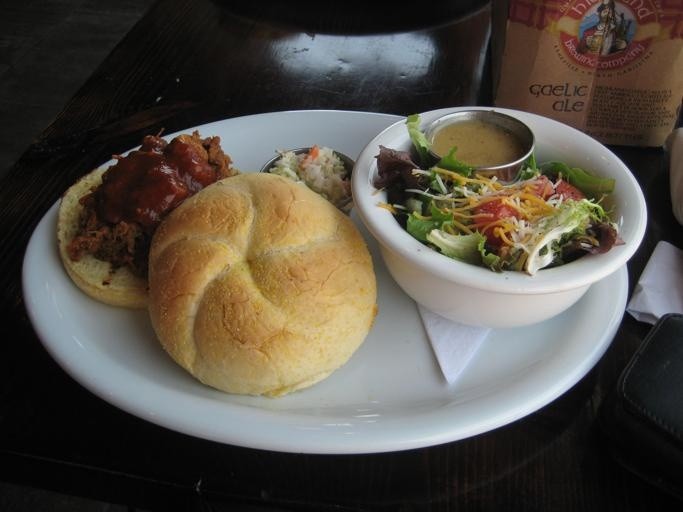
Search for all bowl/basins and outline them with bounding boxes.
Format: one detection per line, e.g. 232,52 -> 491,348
351,106 -> 650,329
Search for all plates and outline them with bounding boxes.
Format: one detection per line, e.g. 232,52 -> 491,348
19,107 -> 629,454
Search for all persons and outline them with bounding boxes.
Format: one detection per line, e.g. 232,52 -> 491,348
578,0 -> 627,56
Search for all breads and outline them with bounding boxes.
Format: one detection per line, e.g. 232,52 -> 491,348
55,169 -> 145,308
146,172 -> 377,396
